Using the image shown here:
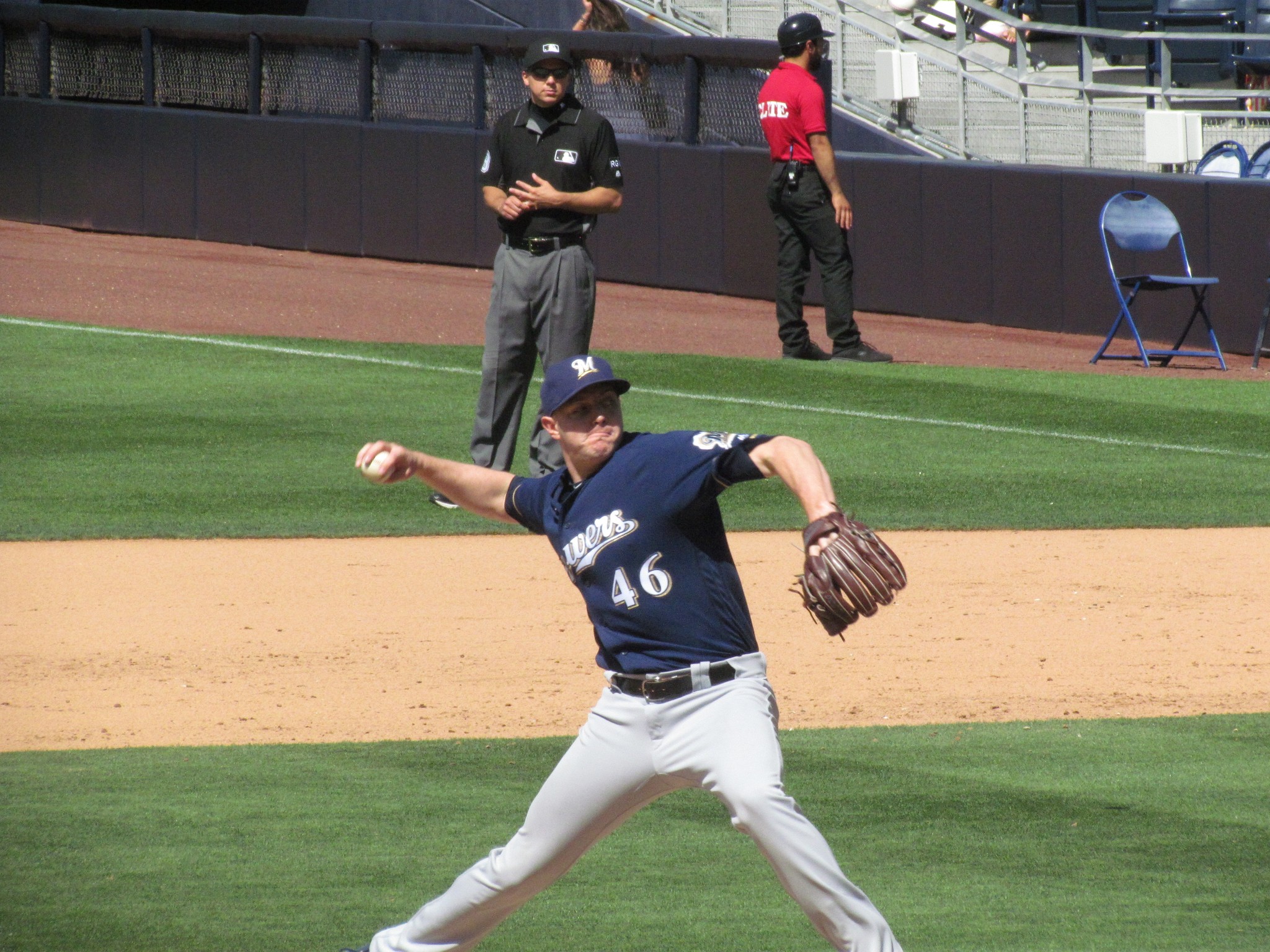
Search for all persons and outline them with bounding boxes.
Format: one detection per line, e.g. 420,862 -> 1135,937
572,0 -> 672,142
429,38 -> 624,507
355,351 -> 909,952
756,13 -> 896,367
982,0 -> 1039,45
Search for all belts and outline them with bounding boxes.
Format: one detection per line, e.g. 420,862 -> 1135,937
612,664 -> 737,701
503,232 -> 586,256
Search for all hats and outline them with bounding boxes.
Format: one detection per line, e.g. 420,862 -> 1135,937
521,35 -> 573,70
541,355 -> 630,415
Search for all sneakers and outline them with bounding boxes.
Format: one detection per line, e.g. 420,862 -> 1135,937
782,341 -> 833,361
833,341 -> 894,363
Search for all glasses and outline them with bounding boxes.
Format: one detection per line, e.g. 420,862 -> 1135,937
531,67 -> 570,79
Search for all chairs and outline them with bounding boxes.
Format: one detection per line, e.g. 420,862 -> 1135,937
1006,0 -> 1270,126
1090,191 -> 1228,369
1195,140 -> 1270,179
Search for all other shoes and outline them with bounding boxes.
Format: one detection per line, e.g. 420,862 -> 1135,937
430,492 -> 456,508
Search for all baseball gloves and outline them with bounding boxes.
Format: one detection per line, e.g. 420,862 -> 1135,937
800,501 -> 908,641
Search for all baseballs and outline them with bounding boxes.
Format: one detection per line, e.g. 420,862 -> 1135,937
360,449 -> 396,484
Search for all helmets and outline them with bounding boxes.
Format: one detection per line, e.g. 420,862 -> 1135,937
778,12 -> 835,46
